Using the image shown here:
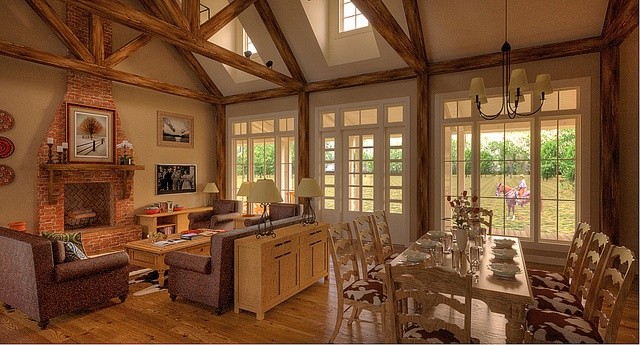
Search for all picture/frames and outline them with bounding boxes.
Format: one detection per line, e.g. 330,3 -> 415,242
157,110 -> 194,148
66,102 -> 116,165
155,163 -> 198,196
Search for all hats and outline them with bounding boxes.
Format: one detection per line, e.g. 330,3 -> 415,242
520,174 -> 524,177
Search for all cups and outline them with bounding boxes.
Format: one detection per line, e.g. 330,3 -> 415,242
480,228 -> 486,243
452,250 -> 459,269
433,247 -> 442,266
444,235 -> 452,254
470,246 -> 478,265
451,226 -> 458,242
475,236 -> 484,253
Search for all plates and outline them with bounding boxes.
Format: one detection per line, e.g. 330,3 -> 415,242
487,263 -> 521,278
401,250 -> 430,262
427,230 -> 446,238
142,209 -> 159,213
173,207 -> 183,211
491,248 -> 518,259
414,239 -> 438,249
494,239 -> 515,248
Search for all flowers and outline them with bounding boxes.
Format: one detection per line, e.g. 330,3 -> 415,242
117,139 -> 134,157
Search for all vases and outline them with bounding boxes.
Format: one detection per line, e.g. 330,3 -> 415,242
120,157 -> 132,164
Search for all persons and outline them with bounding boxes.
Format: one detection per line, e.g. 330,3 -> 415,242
158,166 -> 193,190
516,173 -> 528,206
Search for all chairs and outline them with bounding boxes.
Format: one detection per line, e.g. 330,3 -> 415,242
384,263 -> 480,344
524,244 -> 636,344
526,222 -> 592,292
371,210 -> 401,263
327,223 -> 398,340
526,232 -> 610,314
233,221 -> 330,321
353,216 -> 386,317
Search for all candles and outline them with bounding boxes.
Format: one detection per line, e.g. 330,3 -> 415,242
56,146 -> 63,152
61,143 -> 68,148
45,138 -> 55,143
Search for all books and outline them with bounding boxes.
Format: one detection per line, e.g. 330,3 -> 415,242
180,232 -> 203,240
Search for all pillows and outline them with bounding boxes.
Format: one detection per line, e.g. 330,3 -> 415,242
63,242 -> 87,263
41,232 -> 88,258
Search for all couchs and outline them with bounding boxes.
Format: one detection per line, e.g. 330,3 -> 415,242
164,215 -> 314,315
0,227 -> 129,330
188,200 -> 243,231
244,203 -> 300,228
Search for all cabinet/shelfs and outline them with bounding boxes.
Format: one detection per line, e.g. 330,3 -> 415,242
134,206 -> 214,237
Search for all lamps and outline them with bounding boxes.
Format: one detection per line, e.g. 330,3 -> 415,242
247,179 -> 283,239
294,178 -> 325,225
203,183 -> 219,205
469,0 -> 554,118
236,182 -> 256,196
